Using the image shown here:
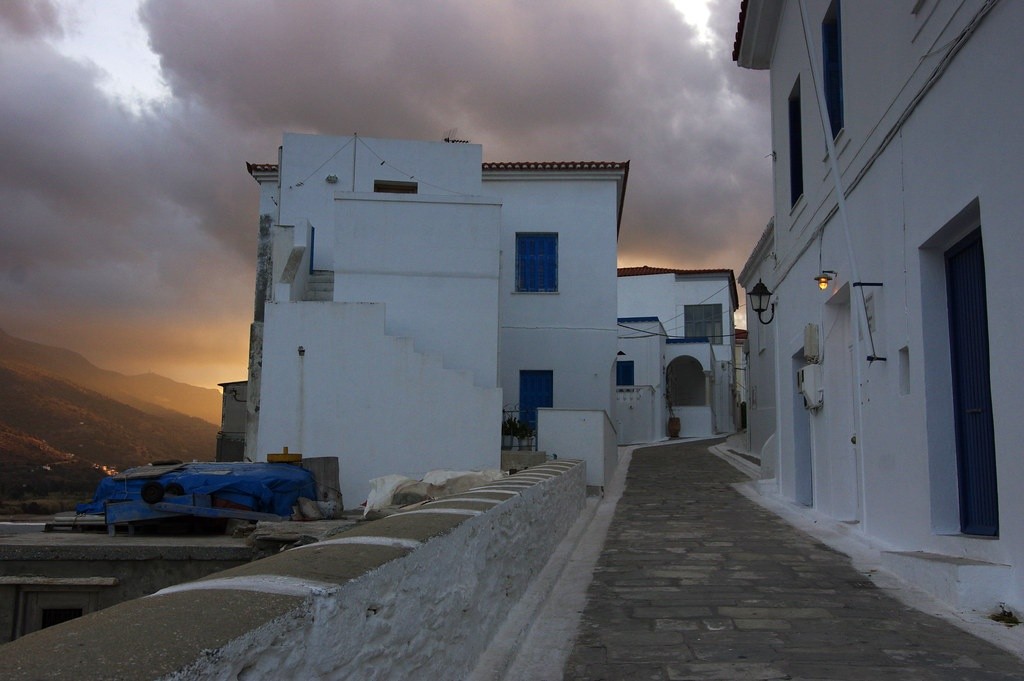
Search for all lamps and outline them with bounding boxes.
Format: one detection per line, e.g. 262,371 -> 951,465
813,270 -> 838,290
746,277 -> 775,324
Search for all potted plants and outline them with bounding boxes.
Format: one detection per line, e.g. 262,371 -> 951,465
502,417 -> 532,451
